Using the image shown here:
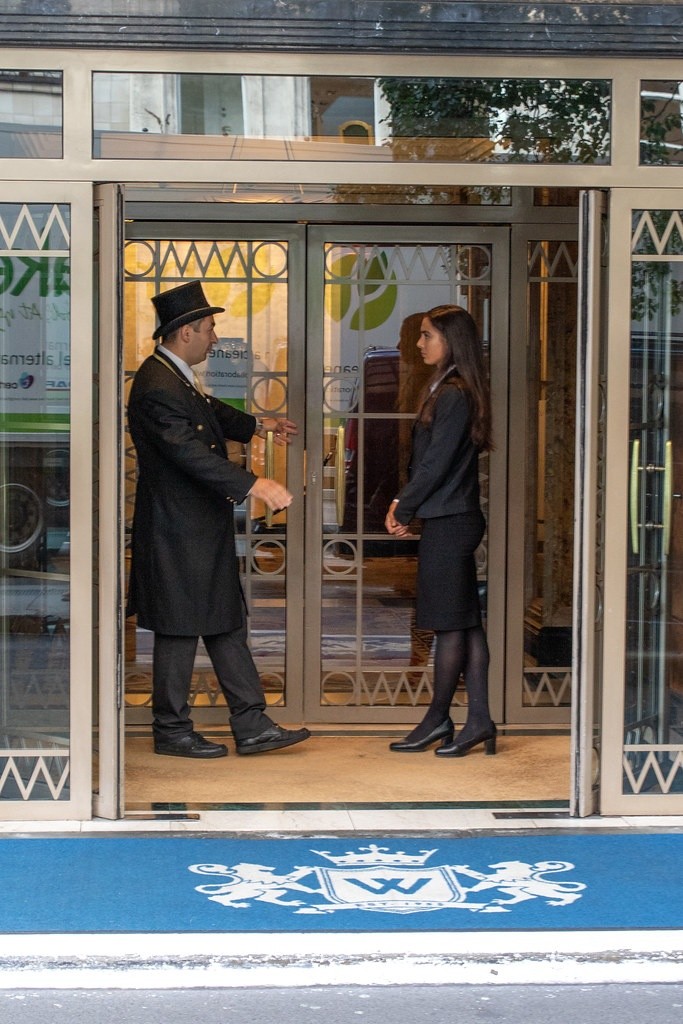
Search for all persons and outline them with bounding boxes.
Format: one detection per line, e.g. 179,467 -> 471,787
385,303 -> 498,759
393,314 -> 436,694
126,280 -> 313,757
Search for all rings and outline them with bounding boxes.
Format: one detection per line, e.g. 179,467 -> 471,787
276,432 -> 281,436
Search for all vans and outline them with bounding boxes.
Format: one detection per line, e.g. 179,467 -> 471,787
340,338 -> 490,609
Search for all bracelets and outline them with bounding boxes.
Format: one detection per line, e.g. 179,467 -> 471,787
253,417 -> 263,436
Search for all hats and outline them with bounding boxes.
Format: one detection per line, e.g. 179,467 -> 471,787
149,279 -> 225,340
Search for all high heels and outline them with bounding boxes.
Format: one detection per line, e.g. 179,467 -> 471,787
390,716 -> 455,752
435,721 -> 498,757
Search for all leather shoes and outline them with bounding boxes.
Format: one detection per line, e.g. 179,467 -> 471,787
236,721 -> 311,754
153,731 -> 227,758
